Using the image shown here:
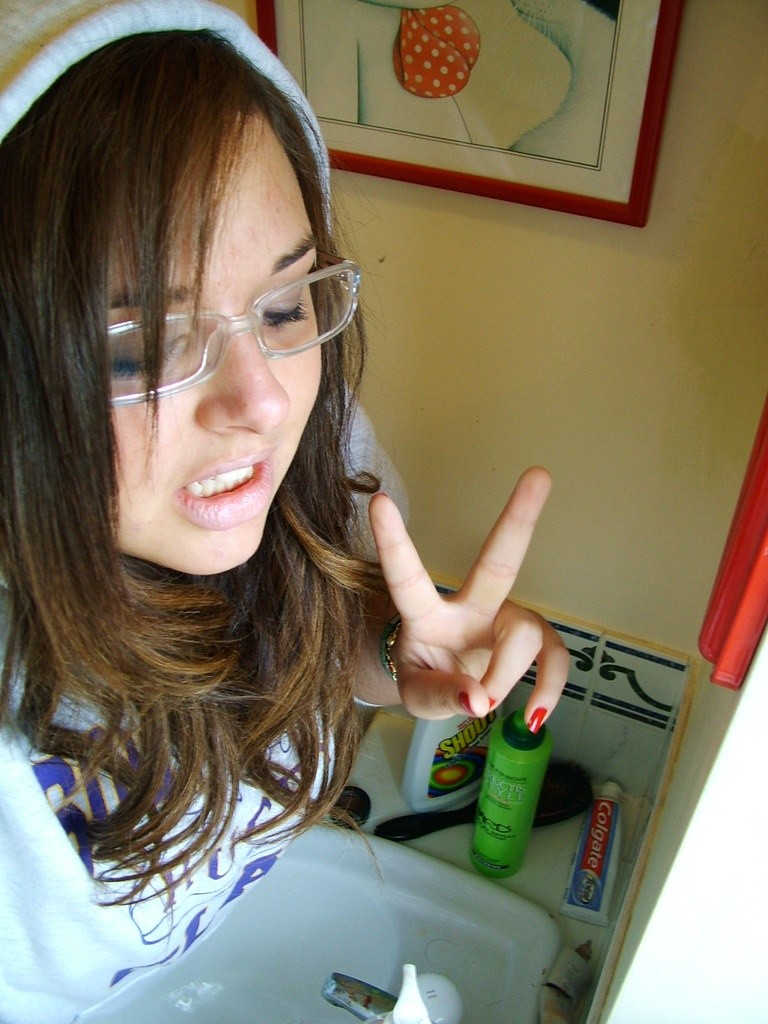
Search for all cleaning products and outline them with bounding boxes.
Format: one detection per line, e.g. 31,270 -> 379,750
399,697 -> 555,880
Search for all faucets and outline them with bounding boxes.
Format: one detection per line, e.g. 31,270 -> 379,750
319,963 -> 468,1024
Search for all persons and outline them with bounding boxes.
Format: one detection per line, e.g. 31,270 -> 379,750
0,0 -> 569,1024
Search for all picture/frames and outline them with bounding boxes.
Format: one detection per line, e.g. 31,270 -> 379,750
255,0 -> 685,228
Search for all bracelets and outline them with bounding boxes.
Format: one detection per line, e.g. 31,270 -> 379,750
380,612 -> 401,682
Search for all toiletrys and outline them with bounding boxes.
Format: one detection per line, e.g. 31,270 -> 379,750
537,938 -> 597,1024
556,779 -> 624,928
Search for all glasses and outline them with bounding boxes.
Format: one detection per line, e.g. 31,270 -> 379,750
104,248 -> 362,407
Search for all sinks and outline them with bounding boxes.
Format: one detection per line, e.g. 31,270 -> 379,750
90,821 -> 566,1024
